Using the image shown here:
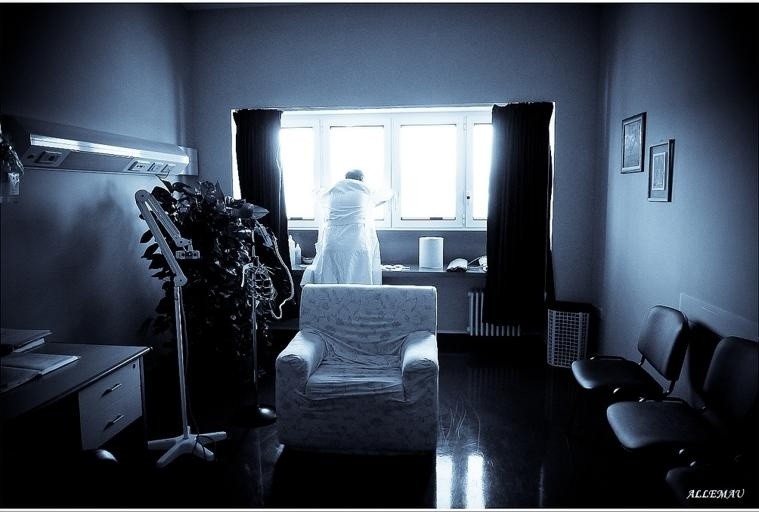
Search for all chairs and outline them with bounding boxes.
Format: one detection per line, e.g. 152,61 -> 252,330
606,322 -> 724,451
665,334 -> 759,508
276,285 -> 438,454
574,305 -> 688,401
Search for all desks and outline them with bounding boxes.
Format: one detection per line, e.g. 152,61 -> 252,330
0,343 -> 152,452
289,262 -> 486,332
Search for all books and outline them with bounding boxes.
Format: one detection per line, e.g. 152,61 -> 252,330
0,326 -> 80,391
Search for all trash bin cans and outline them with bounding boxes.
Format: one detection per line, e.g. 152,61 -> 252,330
548,301 -> 592,368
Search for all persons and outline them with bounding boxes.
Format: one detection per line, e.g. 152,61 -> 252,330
300,169 -> 383,289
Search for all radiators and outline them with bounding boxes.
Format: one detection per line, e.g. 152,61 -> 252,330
468,290 -> 521,336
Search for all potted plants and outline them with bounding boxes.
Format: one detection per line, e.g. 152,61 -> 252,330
138,181 -> 299,409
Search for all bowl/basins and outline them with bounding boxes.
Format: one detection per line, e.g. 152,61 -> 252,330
302,257 -> 312,264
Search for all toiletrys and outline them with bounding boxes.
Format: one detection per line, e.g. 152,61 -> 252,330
288,234 -> 303,266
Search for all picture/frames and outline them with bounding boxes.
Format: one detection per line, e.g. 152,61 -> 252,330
648,139 -> 673,202
621,111 -> 647,174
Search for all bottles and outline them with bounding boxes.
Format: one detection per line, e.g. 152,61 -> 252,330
295,244 -> 301,264
288,235 -> 295,266
